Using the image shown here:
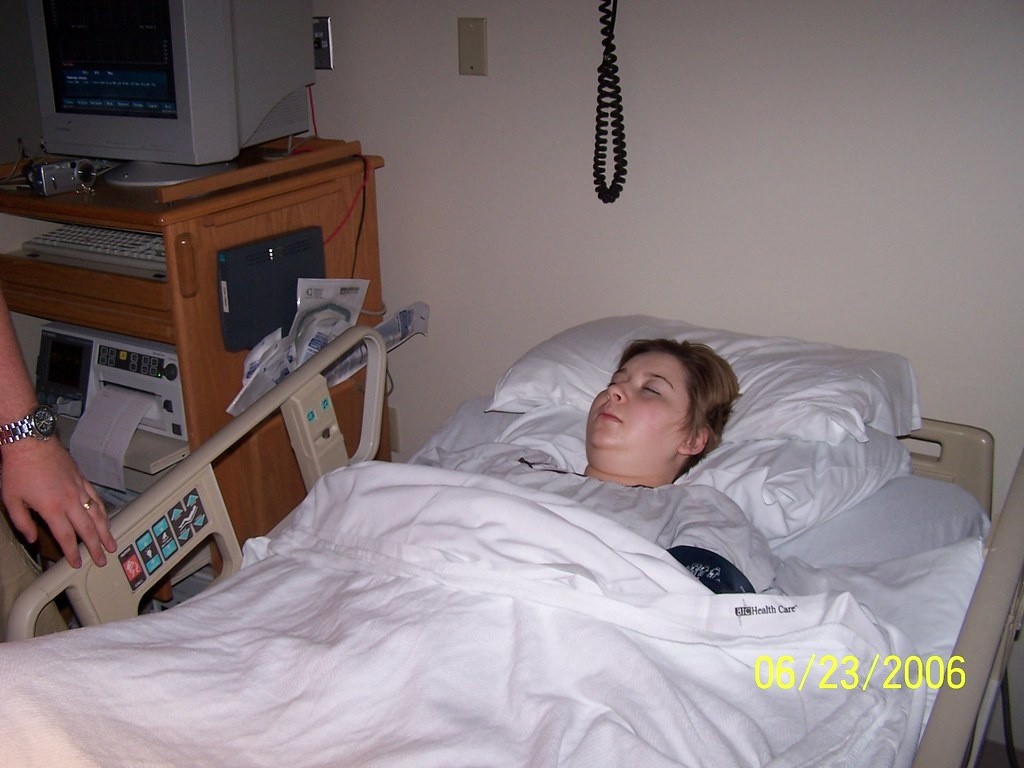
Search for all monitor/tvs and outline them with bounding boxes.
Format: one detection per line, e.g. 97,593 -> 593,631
26,0 -> 316,186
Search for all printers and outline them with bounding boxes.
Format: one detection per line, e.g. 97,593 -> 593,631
33,321 -> 191,494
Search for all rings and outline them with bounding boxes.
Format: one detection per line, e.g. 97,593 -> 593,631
84,498 -> 92,509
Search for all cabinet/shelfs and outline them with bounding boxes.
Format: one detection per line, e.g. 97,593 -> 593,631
1,136 -> 392,630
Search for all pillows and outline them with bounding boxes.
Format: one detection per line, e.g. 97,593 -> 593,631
482,315 -> 922,446
496,404 -> 914,539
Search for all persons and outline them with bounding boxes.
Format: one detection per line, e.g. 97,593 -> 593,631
0,293 -> 118,645
497,339 -> 777,593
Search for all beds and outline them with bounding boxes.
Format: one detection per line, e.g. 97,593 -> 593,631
1,326 -> 1024,768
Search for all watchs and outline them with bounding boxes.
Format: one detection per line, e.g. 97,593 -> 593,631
0,406 -> 59,445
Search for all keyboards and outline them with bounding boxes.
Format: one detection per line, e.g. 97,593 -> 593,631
23,222 -> 167,273
